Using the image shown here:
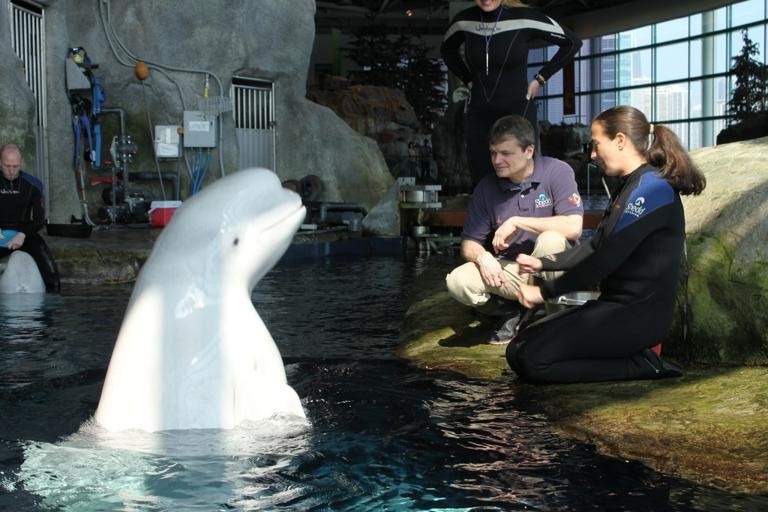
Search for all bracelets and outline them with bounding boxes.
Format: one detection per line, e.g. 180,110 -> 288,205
534,74 -> 546,87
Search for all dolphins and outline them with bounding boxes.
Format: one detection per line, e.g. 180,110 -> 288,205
0,251 -> 47,294
94,166 -> 308,435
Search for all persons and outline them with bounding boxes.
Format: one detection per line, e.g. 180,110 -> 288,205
0,143 -> 61,294
419,139 -> 432,182
506,106 -> 707,385
440,0 -> 583,192
445,115 -> 584,345
408,142 -> 416,178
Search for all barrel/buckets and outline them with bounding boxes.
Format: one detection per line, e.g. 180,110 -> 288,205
539,269 -> 603,316
